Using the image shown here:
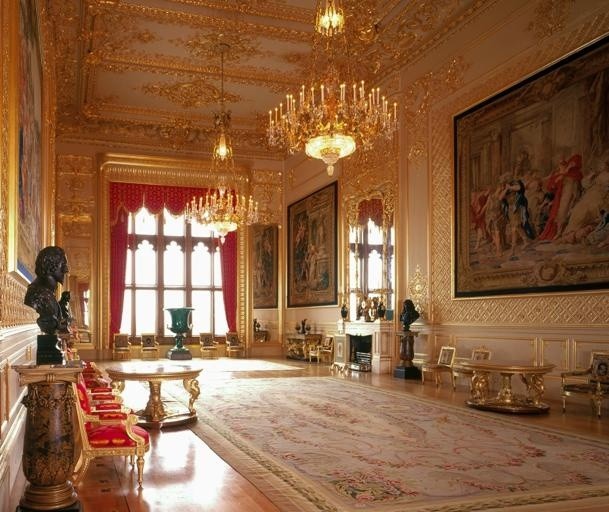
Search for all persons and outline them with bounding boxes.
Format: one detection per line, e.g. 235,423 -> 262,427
205,337 -> 209,345
59,291 -> 71,320
400,300 -> 420,323
252,231 -> 274,305
296,215 -> 329,290
341,296 -> 386,321
146,338 -> 152,346
598,362 -> 607,377
23,246 -> 68,334
469,149 -> 608,257
230,337 -> 235,346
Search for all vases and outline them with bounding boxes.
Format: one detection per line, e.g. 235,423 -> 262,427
163,307 -> 196,360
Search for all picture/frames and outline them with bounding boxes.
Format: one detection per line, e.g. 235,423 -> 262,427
249,221 -> 280,311
285,177 -> 340,311
449,27 -> 609,302
11,86 -> 52,288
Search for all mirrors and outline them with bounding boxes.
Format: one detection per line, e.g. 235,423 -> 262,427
57,211 -> 95,348
342,180 -> 395,318
246,217 -> 284,345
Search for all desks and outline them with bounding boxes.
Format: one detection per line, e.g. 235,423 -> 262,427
455,355 -> 557,419
280,332 -> 317,364
255,330 -> 269,341
106,360 -> 202,432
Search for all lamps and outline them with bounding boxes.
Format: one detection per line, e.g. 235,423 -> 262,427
261,0 -> 402,182
182,43 -> 265,244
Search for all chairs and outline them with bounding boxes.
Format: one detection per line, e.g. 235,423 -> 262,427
453,346 -> 491,396
199,331 -> 219,360
112,332 -> 132,362
308,335 -> 334,366
139,333 -> 160,360
67,349 -> 149,494
560,346 -> 609,418
420,345 -> 456,388
224,331 -> 244,358
